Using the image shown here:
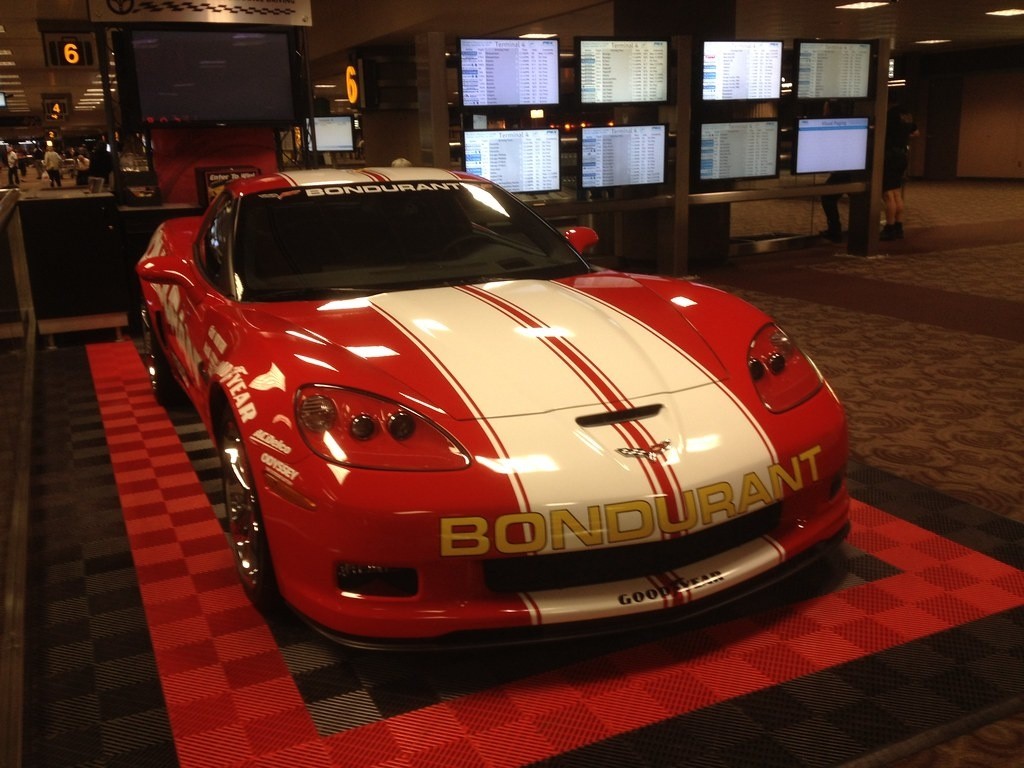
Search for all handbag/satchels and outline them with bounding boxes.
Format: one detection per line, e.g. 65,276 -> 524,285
46,161 -> 55,171
42,171 -> 51,185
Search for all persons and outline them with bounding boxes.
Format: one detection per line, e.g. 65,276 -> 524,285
3,145 -> 29,188
73,141 -> 115,187
879,94 -> 921,241
32,141 -> 66,188
817,171 -> 849,247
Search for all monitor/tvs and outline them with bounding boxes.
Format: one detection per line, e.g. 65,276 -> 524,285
305,115 -> 357,153
122,23 -> 304,127
456,36 -> 877,195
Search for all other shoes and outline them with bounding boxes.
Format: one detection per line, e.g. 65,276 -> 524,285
819,228 -> 844,241
879,222 -> 904,241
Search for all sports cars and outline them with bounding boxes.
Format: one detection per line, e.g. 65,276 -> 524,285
133,166 -> 852,661
